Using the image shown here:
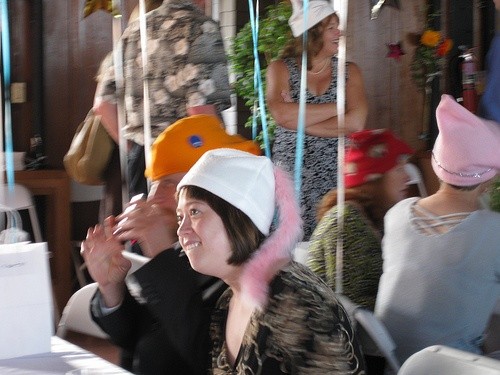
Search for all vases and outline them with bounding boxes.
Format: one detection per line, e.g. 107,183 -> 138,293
417,150 -> 440,197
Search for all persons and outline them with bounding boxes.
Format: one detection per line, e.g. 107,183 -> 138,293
95,0 -> 233,256
266,0 -> 369,242
306,128 -> 416,312
374,93 -> 500,367
175,146 -> 369,375
56,113 -> 261,346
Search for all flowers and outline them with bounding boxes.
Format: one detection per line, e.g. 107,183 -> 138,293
411,32 -> 454,152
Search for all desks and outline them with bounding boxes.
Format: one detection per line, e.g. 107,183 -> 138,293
0,335 -> 136,375
5,169 -> 113,315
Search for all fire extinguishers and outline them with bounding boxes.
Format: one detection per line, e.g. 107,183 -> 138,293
459,47 -> 480,114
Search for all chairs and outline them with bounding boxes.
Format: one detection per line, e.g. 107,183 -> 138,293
0,184 -> 54,260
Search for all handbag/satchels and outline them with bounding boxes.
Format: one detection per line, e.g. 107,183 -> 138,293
63,112 -> 117,186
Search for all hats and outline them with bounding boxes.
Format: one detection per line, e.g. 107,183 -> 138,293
430,95 -> 500,183
176,147 -> 275,237
287,0 -> 335,38
145,114 -> 261,181
343,127 -> 416,188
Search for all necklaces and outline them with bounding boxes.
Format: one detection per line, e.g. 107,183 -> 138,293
305,61 -> 329,75
